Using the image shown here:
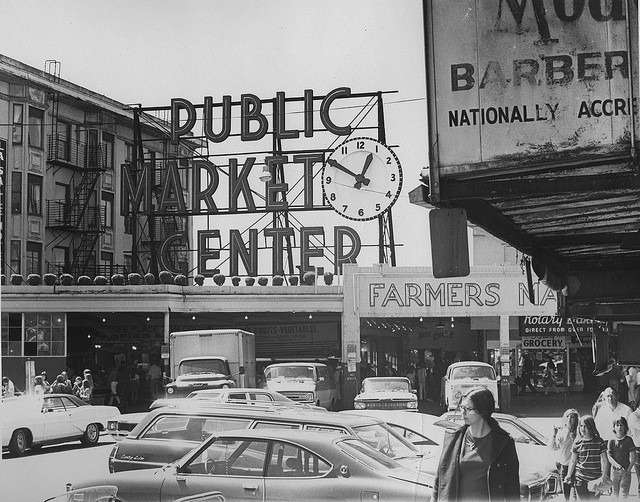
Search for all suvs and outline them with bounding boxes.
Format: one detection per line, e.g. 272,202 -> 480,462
109,405 -> 439,475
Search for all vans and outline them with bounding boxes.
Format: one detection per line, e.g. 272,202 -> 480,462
260,362 -> 337,412
444,360 -> 498,411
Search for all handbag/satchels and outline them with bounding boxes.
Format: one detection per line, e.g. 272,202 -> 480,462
587,475 -> 613,497
513,376 -> 523,386
540,375 -> 554,388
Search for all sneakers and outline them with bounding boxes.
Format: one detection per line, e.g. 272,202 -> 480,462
543,393 -> 548,396
555,391 -> 560,395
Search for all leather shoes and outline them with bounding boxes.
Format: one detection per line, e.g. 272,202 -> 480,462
520,390 -> 526,393
535,393 -> 540,398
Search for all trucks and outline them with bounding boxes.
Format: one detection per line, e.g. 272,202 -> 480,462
165,329 -> 255,399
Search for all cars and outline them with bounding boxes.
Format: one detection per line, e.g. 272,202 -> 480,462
66,430 -> 435,502
339,410 -> 562,494
354,377 -> 419,413
0,394 -> 121,457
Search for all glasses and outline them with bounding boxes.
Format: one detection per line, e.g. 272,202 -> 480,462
459,405 -> 477,413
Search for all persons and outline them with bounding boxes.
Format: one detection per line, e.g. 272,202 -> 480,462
2,377 -> 14,398
34,366 -> 95,404
554,409 -> 581,502
367,363 -> 427,401
518,352 -> 539,397
104,358 -> 161,406
543,356 -> 560,396
629,400 -> 640,492
606,417 -> 636,502
427,386 -> 523,502
592,386 -> 634,477
563,415 -> 610,502
